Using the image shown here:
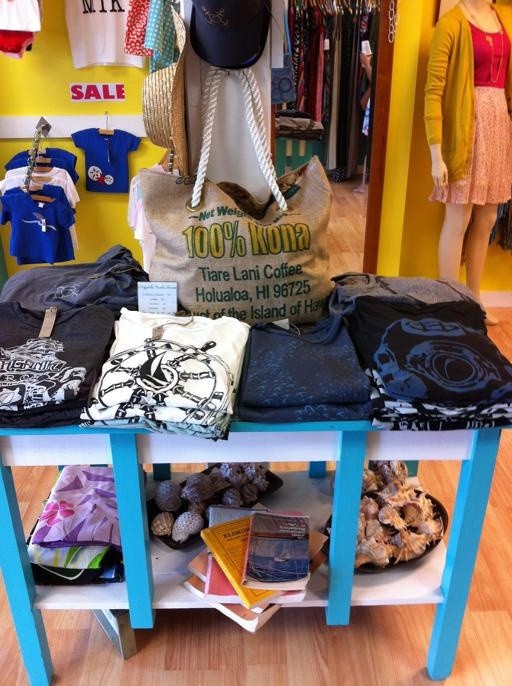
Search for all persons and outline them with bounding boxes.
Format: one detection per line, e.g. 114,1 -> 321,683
420,0 -> 511,324
359,52 -> 374,137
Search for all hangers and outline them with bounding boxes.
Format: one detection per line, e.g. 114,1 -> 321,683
291,0 -> 381,17
21,130 -> 56,203
99,110 -> 115,136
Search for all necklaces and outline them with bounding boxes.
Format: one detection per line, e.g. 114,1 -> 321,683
461,2 -> 505,83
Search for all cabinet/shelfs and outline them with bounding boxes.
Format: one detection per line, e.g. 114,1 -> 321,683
0,422 -> 512,686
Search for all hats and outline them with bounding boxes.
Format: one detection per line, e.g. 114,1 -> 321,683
189,0 -> 271,69
141,5 -> 190,178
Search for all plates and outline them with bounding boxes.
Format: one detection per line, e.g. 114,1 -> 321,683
321,488 -> 449,571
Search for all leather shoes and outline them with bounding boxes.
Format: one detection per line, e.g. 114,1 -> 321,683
479,304 -> 499,326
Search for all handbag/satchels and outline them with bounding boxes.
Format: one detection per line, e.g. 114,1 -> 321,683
138,66 -> 334,327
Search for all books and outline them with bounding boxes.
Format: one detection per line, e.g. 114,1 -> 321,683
182,506 -> 329,633
